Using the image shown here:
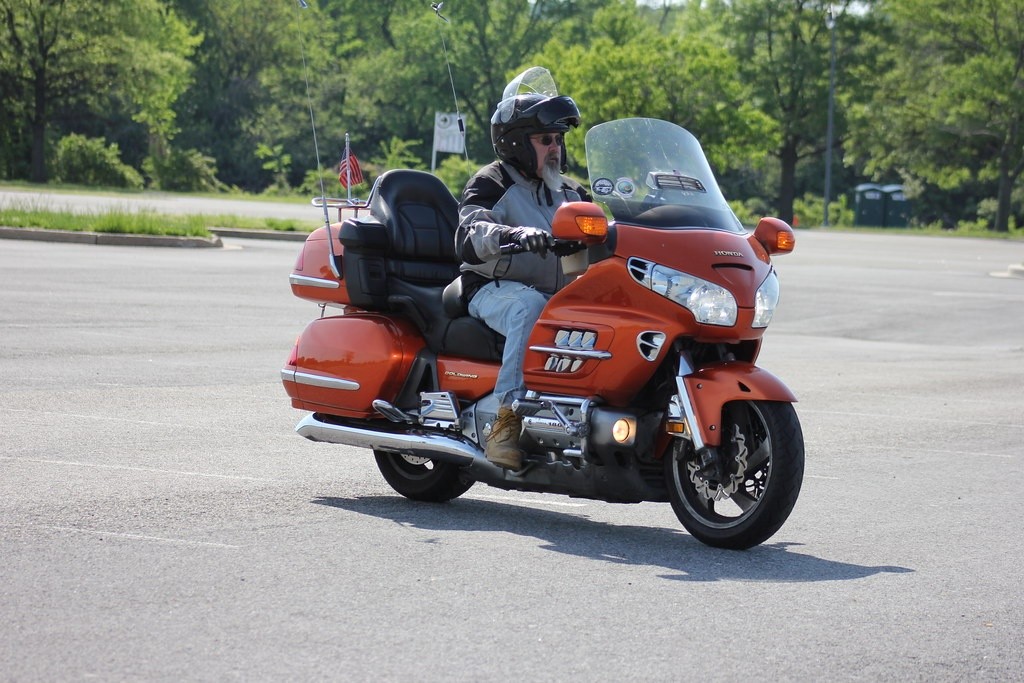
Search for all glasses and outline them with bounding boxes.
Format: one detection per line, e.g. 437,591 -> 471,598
529,134 -> 563,145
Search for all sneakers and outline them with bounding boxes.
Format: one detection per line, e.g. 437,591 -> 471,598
484,408 -> 523,470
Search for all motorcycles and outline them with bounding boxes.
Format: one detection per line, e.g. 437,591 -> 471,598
274,108 -> 806,551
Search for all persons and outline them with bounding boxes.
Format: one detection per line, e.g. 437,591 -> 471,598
454,66 -> 608,468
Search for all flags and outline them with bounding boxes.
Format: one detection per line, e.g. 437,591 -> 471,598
338,147 -> 363,188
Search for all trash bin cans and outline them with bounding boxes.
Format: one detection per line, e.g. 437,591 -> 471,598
852,183 -> 886,229
883,184 -> 914,228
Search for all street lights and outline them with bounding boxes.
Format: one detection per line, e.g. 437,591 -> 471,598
819,1 -> 839,227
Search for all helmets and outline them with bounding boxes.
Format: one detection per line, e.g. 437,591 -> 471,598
490,92 -> 581,174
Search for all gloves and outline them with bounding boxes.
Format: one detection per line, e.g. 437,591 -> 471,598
500,226 -> 555,259
